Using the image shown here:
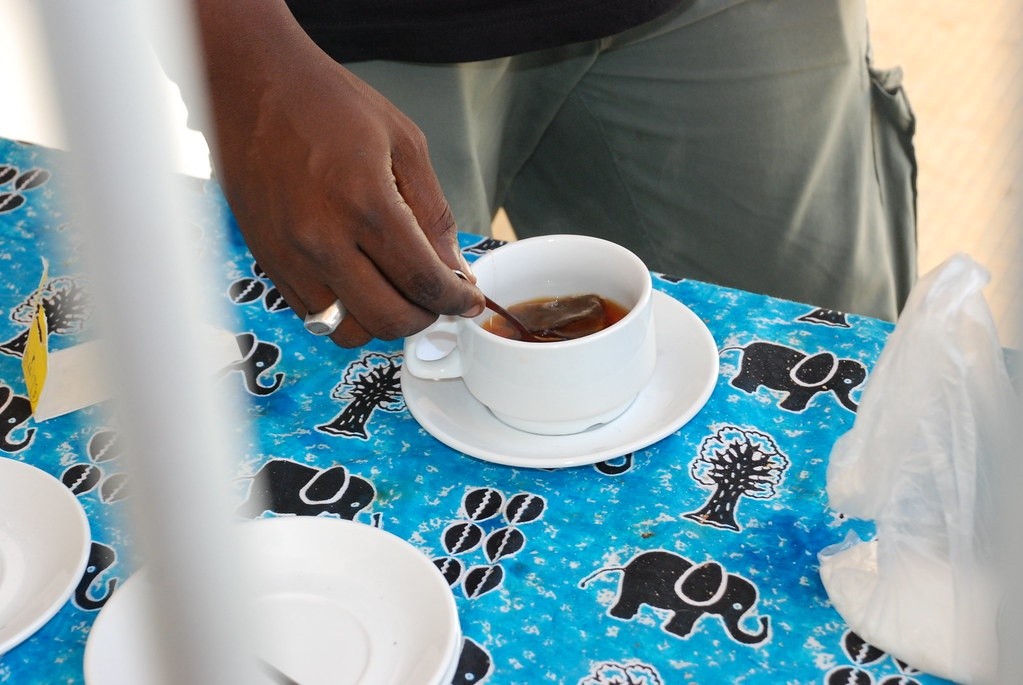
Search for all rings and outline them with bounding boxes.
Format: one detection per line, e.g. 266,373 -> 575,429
304,297 -> 348,337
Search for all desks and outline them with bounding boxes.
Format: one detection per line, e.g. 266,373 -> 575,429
1,134 -> 971,685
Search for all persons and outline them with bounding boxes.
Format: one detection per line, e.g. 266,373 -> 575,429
166,0 -> 921,352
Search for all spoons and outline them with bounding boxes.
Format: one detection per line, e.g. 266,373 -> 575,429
453,268 -> 567,342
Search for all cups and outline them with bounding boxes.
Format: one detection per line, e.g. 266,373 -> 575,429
404,234 -> 658,437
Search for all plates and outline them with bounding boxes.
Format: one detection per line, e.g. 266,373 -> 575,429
400,289 -> 721,469
0,456 -> 93,658
82,515 -> 464,685
